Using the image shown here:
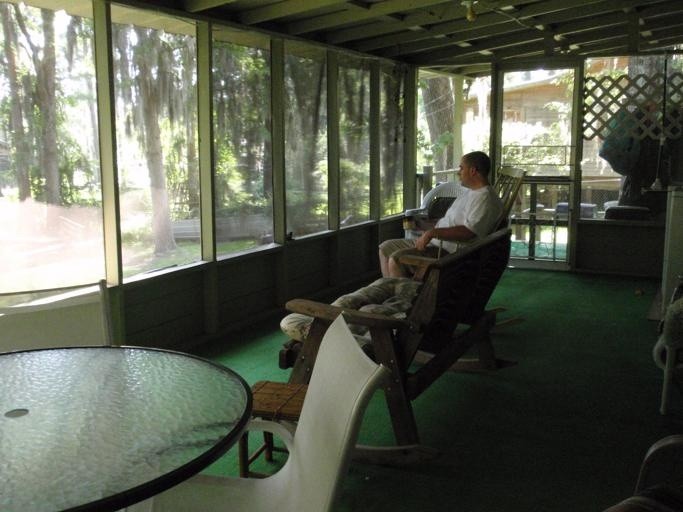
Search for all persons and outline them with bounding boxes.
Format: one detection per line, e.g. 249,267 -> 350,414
377,151 -> 503,281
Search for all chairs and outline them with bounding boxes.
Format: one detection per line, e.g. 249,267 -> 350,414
601,300 -> 683,512
401,166 -> 525,235
148,308 -> 389,510
394,207 -> 510,328
405,183 -> 475,254
2,278 -> 134,355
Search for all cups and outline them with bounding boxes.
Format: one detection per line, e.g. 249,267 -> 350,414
402,215 -> 417,230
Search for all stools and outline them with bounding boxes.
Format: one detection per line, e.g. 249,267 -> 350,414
535,201 -> 597,220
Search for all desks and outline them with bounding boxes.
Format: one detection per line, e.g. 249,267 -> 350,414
0,343 -> 251,511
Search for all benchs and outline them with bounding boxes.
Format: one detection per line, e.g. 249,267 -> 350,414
239,228 -> 516,495
